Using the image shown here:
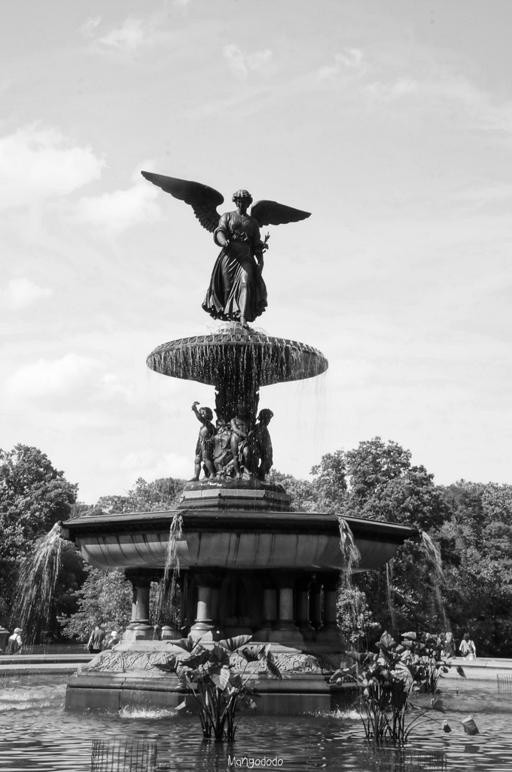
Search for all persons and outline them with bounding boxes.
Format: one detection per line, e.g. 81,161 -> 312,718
101,630 -> 119,649
444,632 -> 465,678
141,170 -> 312,330
191,401 -> 275,481
88,626 -> 104,654
6,628 -> 23,655
458,633 -> 476,660
